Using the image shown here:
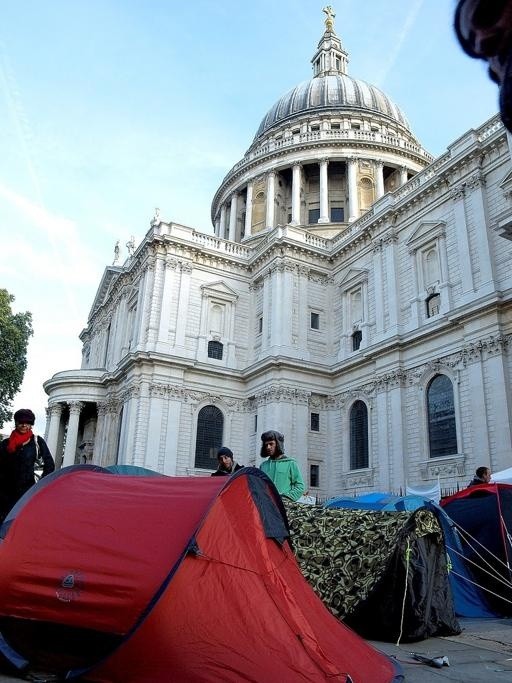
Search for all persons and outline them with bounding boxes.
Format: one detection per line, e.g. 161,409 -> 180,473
258,429 -> 306,502
454,0 -> 512,137
211,444 -> 244,477
1,407 -> 55,518
467,464 -> 492,488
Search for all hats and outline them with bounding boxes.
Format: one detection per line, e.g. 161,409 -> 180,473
217,447 -> 233,460
260,431 -> 284,457
14,409 -> 35,425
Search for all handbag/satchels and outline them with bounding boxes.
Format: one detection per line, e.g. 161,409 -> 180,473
33,456 -> 45,484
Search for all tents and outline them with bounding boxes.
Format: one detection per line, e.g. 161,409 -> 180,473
279,494 -> 464,645
437,481 -> 511,622
326,493 -> 495,620
0,457 -> 167,547
1,463 -> 406,683
